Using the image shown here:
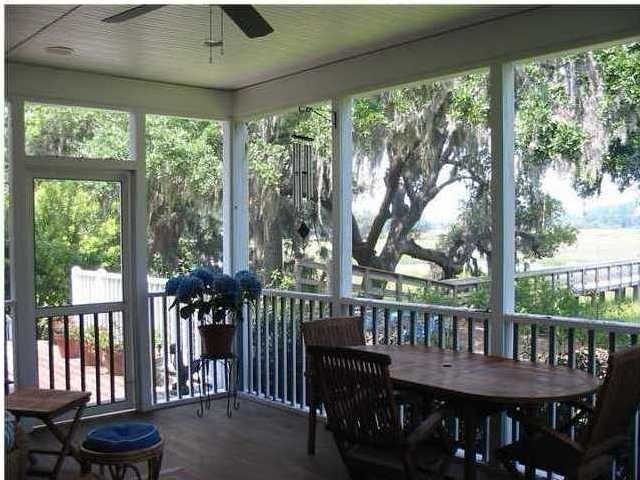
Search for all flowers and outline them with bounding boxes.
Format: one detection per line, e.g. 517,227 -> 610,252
163,262 -> 263,324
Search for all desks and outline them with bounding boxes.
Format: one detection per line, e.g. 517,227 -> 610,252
321,346 -> 603,477
6,388 -> 92,480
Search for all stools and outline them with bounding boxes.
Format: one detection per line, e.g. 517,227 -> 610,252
81,421 -> 167,479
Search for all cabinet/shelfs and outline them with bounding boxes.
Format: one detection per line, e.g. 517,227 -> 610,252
197,356 -> 241,419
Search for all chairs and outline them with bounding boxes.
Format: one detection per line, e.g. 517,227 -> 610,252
302,318 -> 366,457
305,345 -> 464,477
497,344 -> 640,478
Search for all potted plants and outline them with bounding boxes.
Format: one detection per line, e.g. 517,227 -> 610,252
103,322 -> 124,377
51,319 -> 80,360
80,324 -> 107,365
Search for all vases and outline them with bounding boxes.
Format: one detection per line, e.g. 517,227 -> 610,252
203,324 -> 234,353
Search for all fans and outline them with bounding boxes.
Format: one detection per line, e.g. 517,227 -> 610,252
101,4 -> 275,41
